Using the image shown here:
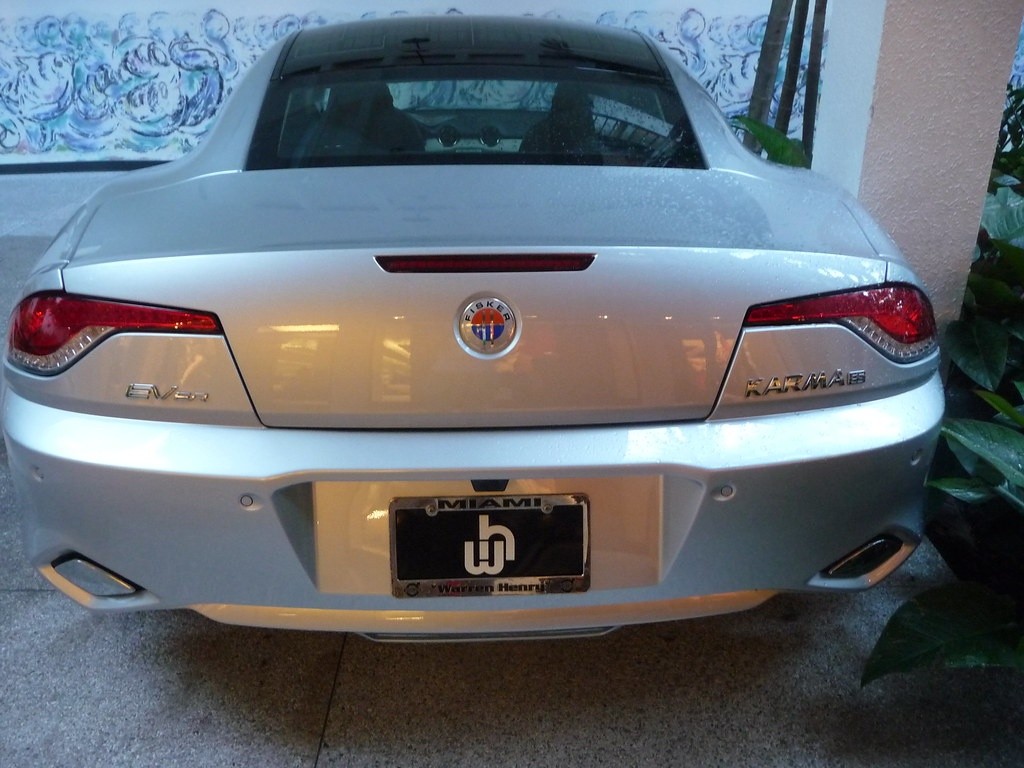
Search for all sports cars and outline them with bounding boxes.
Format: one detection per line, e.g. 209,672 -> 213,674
0,13 -> 947,643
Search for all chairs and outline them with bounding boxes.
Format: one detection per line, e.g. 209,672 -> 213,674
524,78 -> 629,166
301,83 -> 424,162
